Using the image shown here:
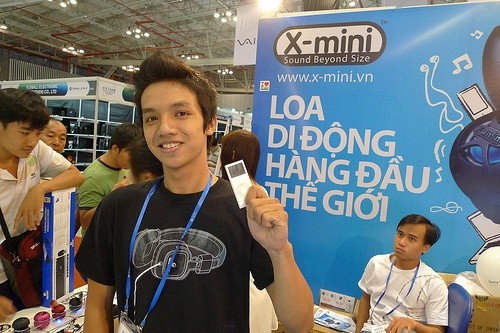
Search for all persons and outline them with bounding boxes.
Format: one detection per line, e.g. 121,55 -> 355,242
219,130 -> 278,333
128,136 -> 164,183
0,88 -> 84,321
207,138 -> 221,175
75,122 -> 144,236
355,214 -> 449,333
39,117 -> 67,154
74,54 -> 316,333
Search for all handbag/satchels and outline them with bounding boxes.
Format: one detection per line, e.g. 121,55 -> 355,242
0,218 -> 42,308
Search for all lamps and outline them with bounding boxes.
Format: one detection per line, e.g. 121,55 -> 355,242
215,7 -> 236,24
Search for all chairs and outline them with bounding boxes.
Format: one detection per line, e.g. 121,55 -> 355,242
445,283 -> 474,333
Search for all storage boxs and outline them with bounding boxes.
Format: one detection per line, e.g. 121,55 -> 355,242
42,188 -> 76,307
437,273 -> 500,333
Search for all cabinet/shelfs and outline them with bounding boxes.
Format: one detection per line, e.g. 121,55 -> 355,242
43,98 -> 134,171
